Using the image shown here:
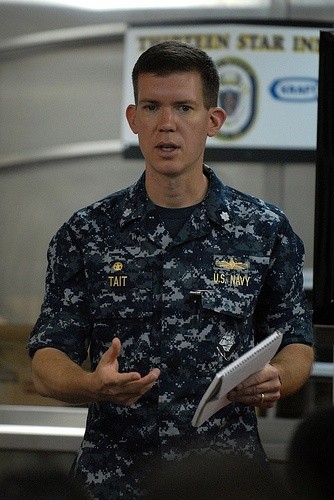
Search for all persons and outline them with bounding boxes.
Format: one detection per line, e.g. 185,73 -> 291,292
0,466 -> 92,500
147,453 -> 257,500
285,408 -> 334,500
30,44 -> 315,500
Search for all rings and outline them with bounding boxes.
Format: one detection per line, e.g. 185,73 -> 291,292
259,393 -> 265,404
